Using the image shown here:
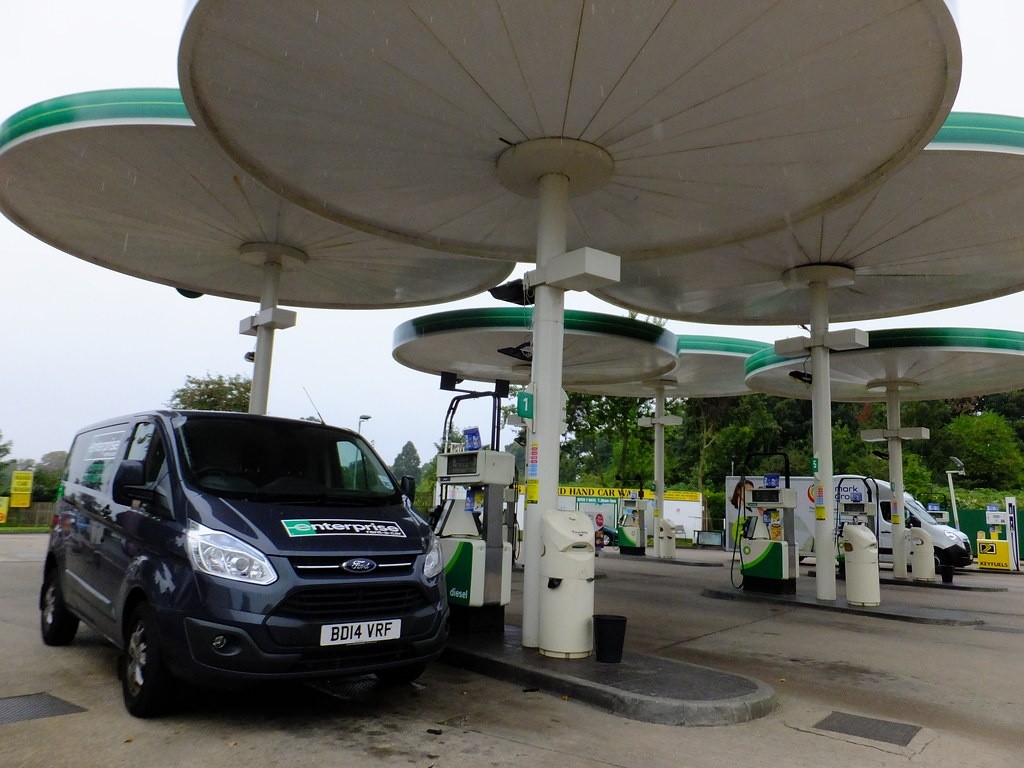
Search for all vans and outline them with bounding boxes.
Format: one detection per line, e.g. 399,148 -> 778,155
725,475 -> 972,584
38,406 -> 453,726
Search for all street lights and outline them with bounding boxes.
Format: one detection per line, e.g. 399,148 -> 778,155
353,415 -> 371,491
